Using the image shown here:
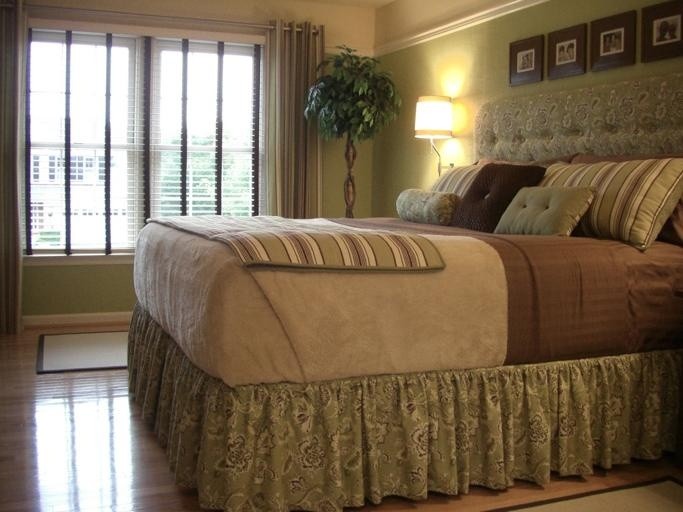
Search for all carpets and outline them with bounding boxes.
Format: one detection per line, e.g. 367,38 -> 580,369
485,476 -> 683,512
35,330 -> 128,374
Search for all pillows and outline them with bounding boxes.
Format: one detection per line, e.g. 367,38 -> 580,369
447,163 -> 547,234
430,164 -> 485,200
395,188 -> 462,226
473,158 -> 557,170
537,157 -> 683,253
493,185 -> 597,237
658,199 -> 683,244
570,152 -> 683,248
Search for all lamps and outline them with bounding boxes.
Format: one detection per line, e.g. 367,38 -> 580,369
413,95 -> 453,179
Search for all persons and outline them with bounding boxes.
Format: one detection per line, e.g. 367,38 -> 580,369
608,35 -> 619,52
566,44 -> 574,61
656,21 -> 669,42
603,39 -> 610,52
520,56 -> 531,70
669,25 -> 677,40
558,46 -> 568,62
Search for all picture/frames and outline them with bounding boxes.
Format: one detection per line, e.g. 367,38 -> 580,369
641,0 -> 683,63
508,34 -> 544,87
590,10 -> 636,72
547,23 -> 587,80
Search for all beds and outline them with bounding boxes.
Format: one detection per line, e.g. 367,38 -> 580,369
127,78 -> 683,512
